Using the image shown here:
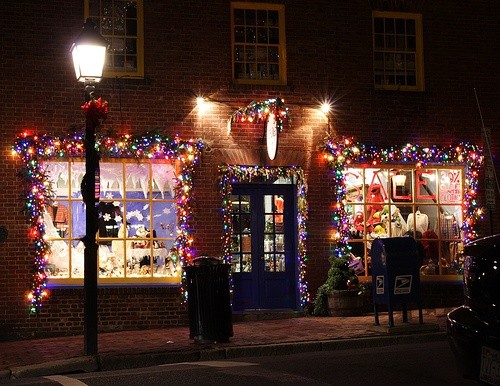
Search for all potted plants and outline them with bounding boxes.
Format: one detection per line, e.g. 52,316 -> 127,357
324,256 -> 360,316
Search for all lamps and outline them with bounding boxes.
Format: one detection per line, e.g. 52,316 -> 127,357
394,175 -> 406,186
266,112 -> 278,160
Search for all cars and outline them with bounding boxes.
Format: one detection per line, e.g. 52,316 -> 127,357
443,234 -> 499,386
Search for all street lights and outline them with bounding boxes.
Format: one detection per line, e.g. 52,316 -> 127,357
68,19 -> 110,356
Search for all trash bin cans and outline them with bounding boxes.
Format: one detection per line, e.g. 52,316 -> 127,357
182,255 -> 235,344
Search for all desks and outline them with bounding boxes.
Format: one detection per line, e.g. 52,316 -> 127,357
129,248 -> 168,269
